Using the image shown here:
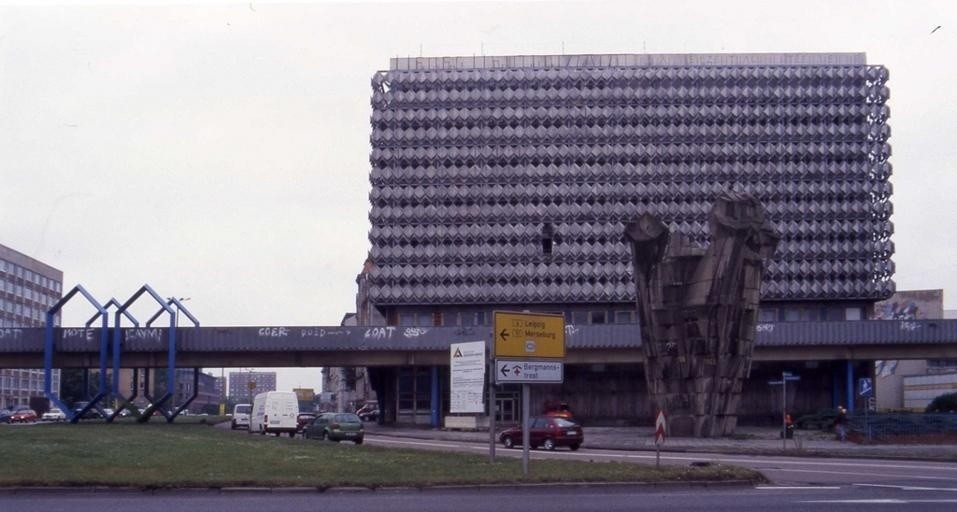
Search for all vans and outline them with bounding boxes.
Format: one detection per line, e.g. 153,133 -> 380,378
355,400 -> 380,416
232,403 -> 253,430
249,390 -> 300,440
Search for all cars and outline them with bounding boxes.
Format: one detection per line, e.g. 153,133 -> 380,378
499,415 -> 585,451
0,401 -> 200,425
359,409 -> 382,422
302,411 -> 366,445
544,399 -> 575,419
296,410 -> 316,432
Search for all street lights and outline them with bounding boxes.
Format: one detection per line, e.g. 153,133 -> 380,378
165,294 -> 192,328
244,367 -> 256,381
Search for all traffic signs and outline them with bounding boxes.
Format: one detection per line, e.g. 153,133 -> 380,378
493,310 -> 567,359
495,358 -> 563,382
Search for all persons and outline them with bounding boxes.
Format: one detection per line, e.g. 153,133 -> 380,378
785,415 -> 794,437
834,409 -> 848,440
